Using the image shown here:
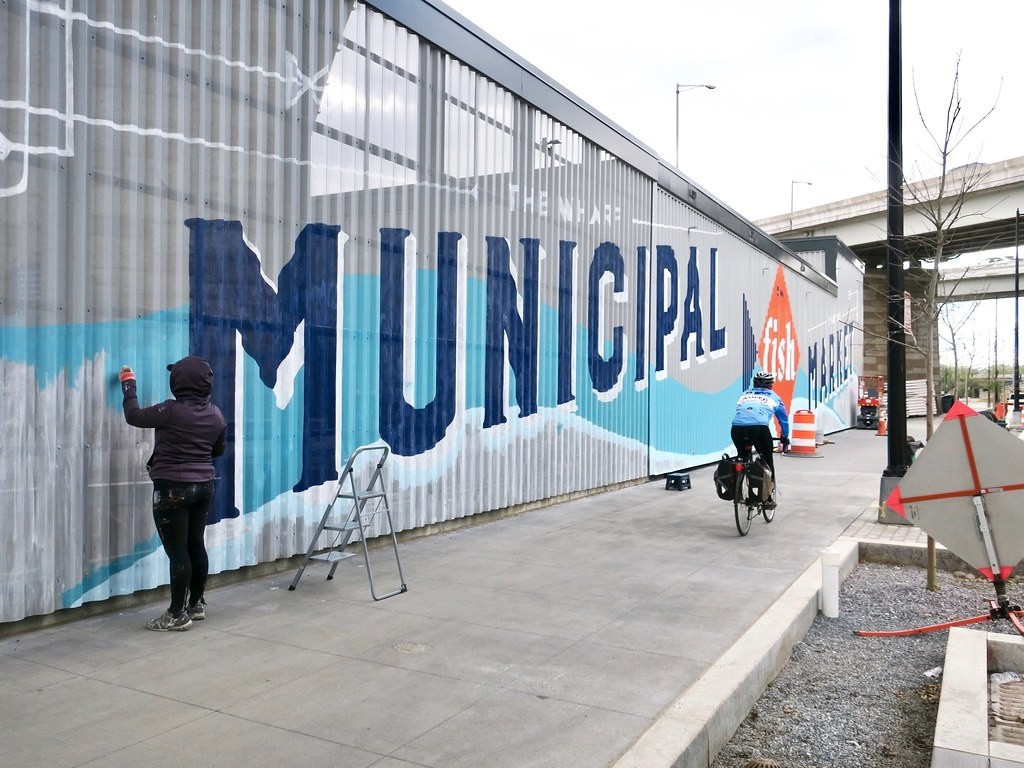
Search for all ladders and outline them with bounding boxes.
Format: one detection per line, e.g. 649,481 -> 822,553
288,446 -> 408,601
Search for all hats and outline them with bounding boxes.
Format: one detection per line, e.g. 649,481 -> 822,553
167,363 -> 174,369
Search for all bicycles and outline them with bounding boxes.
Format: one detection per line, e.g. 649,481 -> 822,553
730,435 -> 791,536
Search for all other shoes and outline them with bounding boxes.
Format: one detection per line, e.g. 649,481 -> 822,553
764,501 -> 778,511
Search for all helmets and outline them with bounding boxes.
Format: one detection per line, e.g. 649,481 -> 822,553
754,372 -> 773,388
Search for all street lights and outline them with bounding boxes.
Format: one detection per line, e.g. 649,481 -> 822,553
789,179 -> 813,237
673,82 -> 716,174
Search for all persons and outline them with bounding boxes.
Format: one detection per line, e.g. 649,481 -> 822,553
730,372 -> 790,508
118,357 -> 230,630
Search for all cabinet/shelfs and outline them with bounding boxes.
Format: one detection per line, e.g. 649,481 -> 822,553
289,443 -> 408,602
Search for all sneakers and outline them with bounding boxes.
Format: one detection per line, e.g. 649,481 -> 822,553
145,602 -> 205,632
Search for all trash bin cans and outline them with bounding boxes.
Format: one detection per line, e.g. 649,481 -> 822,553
941,395 -> 954,413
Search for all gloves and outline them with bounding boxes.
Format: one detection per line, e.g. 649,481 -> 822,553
119,366 -> 136,382
780,434 -> 791,446
